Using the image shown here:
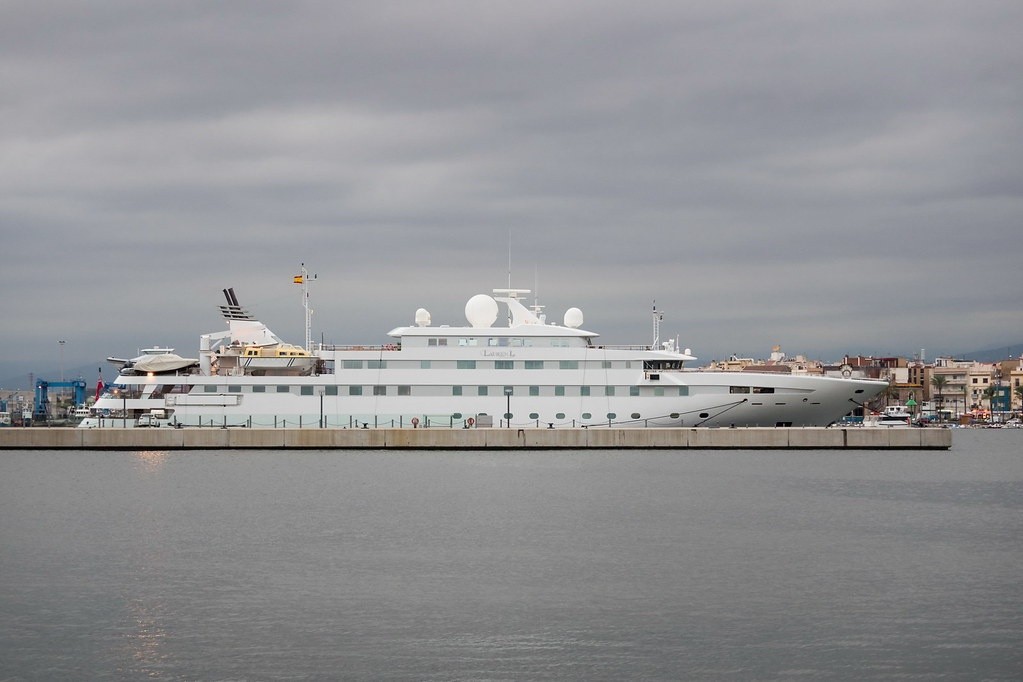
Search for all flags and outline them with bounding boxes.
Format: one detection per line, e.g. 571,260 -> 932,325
294,276 -> 303,283
95,377 -> 104,400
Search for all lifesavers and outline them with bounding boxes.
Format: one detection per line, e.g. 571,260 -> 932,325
411,418 -> 419,425
843,369 -> 851,378
468,417 -> 474,425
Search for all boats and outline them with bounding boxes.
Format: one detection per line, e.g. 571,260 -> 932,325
863,405 -> 911,426
240,344 -> 319,372
74,403 -> 91,418
939,423 -> 1023,429
79,264 -> 890,429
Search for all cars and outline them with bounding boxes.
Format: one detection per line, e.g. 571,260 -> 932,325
138,413 -> 161,428
1006,419 -> 1022,424
916,418 -> 931,425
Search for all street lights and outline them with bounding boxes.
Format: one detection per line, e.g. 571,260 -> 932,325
57,340 -> 67,397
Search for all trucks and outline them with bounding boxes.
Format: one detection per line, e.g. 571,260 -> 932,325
987,416 -> 1000,424
